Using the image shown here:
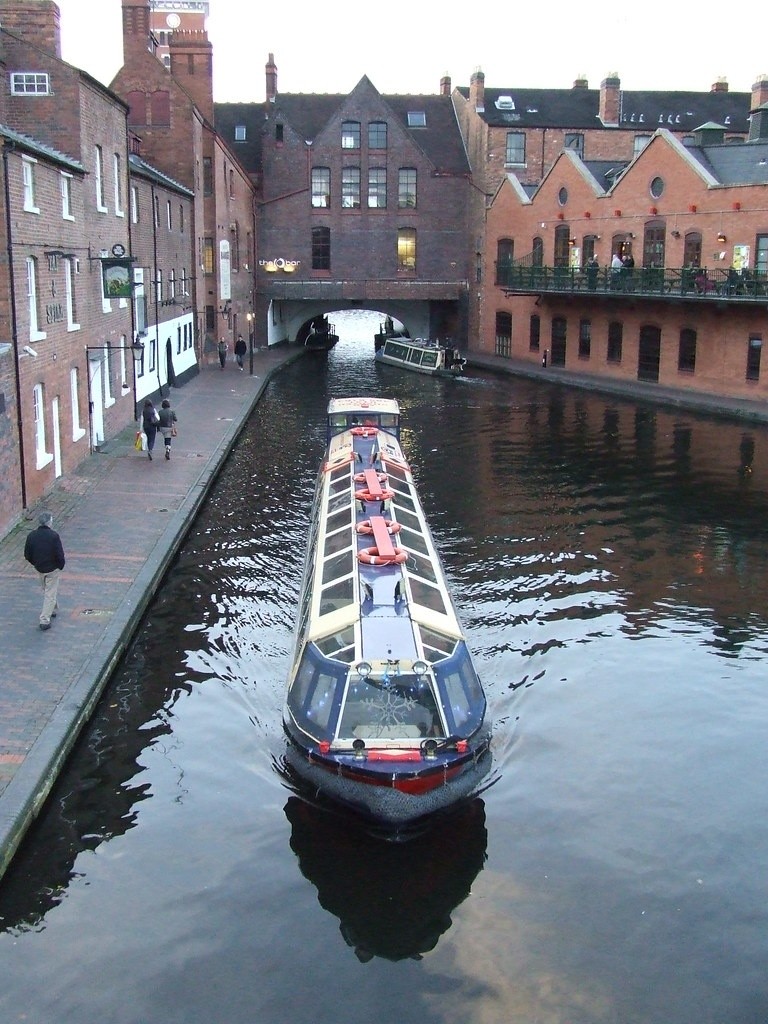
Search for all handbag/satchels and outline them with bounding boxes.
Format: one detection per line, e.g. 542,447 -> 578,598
171,427 -> 178,437
134,431 -> 147,452
150,415 -> 160,426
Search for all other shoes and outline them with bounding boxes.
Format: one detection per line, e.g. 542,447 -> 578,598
165,450 -> 170,460
52,611 -> 56,617
241,368 -> 244,370
148,451 -> 153,461
238,366 -> 241,369
221,367 -> 225,369
39,623 -> 51,630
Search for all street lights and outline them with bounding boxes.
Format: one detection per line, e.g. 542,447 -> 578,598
247,312 -> 255,373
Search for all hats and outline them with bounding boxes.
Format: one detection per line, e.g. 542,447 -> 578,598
239,335 -> 242,337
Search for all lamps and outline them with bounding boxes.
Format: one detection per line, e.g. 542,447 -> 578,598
84,338 -> 145,360
568,239 -> 576,246
593,235 -> 601,241
717,235 -> 726,243
671,230 -> 680,238
626,233 -> 636,240
195,306 -> 230,319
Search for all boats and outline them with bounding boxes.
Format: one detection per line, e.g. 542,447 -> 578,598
282,397 -> 497,828
375,335 -> 467,378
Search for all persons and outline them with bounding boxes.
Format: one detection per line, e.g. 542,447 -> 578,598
140,399 -> 160,461
217,336 -> 228,369
24,511 -> 66,630
610,254 -> 634,290
234,335 -> 247,372
157,400 -> 178,460
584,257 -> 599,290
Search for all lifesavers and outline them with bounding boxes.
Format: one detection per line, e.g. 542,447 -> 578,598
355,488 -> 395,501
355,519 -> 400,535
357,547 -> 409,564
351,427 -> 379,435
352,472 -> 387,482
